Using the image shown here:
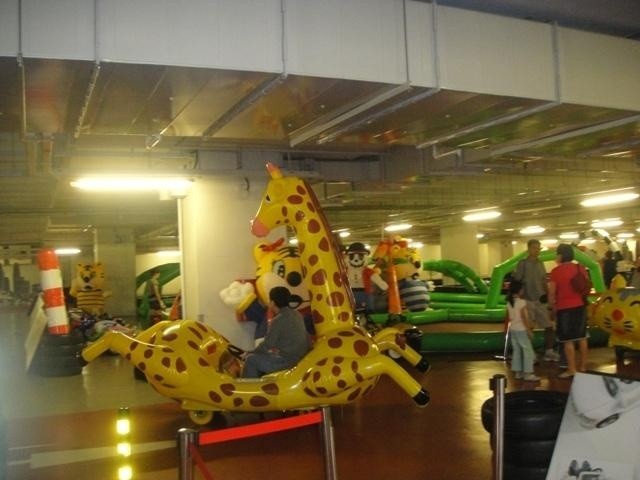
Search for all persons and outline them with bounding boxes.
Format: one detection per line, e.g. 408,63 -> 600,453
146,266 -> 169,327
240,288 -> 310,381
614,251 -> 625,264
506,280 -> 544,382
552,243 -> 592,379
601,250 -> 616,289
400,275 -> 431,313
515,239 -> 562,363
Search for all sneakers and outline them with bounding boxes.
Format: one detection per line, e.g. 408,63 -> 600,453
514,371 -> 541,382
542,351 -> 561,362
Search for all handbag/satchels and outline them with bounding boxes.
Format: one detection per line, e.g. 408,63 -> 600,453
570,272 -> 588,293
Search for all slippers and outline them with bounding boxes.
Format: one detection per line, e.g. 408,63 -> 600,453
557,371 -> 577,378
